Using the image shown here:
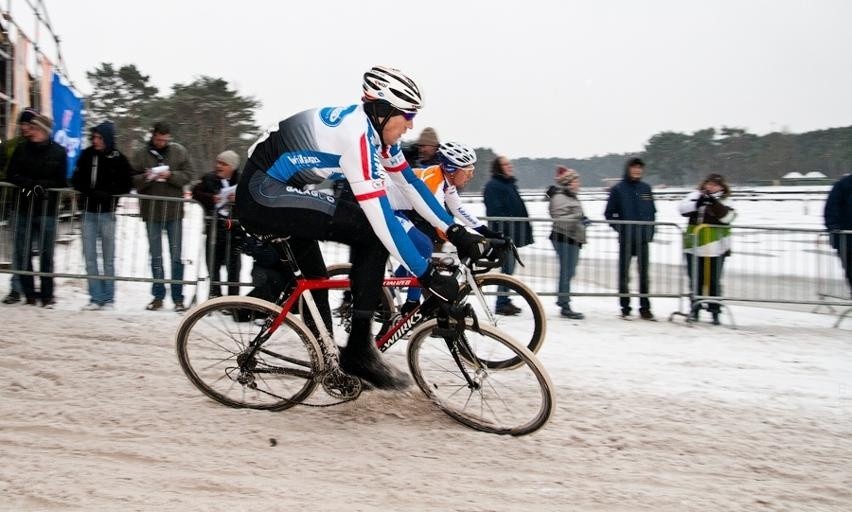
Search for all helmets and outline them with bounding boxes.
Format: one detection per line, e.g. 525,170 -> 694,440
437,141 -> 476,172
362,67 -> 422,116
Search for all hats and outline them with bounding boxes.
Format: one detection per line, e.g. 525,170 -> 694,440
417,127 -> 439,146
18,107 -> 52,136
554,166 -> 579,185
216,150 -> 241,171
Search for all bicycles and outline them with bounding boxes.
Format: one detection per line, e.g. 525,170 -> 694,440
296,226 -> 547,374
177,225 -> 555,435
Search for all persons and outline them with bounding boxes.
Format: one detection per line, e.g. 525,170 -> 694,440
73,123 -> 131,311
681,174 -> 735,325
605,159 -> 656,321
0,107 -> 40,304
825,175 -> 852,301
388,127 -> 504,318
235,66 -> 484,390
483,157 -> 534,315
128,123 -> 192,312
192,150 -> 242,300
5,116 -> 66,305
547,166 -> 589,319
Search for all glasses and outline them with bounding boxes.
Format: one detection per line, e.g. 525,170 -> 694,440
440,159 -> 475,177
216,161 -> 229,166
366,95 -> 416,121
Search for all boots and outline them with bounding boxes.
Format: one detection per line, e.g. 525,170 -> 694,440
339,310 -> 413,391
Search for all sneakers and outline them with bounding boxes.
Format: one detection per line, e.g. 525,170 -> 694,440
400,299 -> 419,316
4,290 -> 57,308
561,304 -> 583,319
146,299 -> 186,311
81,301 -> 115,310
691,310 -> 720,323
495,300 -> 522,315
332,290 -> 353,317
622,313 -> 658,321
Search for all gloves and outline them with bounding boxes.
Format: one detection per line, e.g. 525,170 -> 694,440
21,185 -> 49,200
446,224 -> 506,260
696,194 -> 728,217
417,263 -> 459,306
